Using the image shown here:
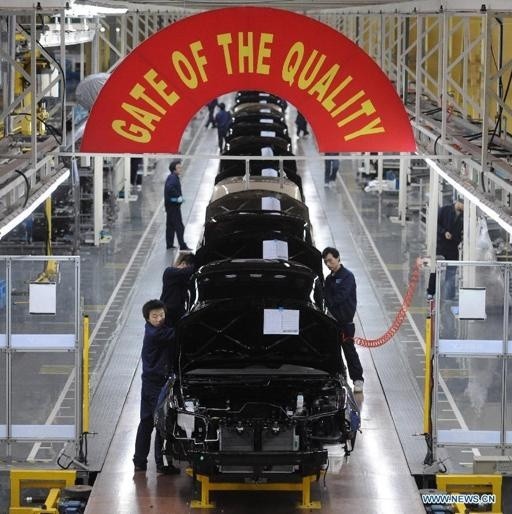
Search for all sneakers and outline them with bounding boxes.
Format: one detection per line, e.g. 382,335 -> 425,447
426,295 -> 433,302
135,463 -> 147,470
167,245 -> 193,250
325,181 -> 336,188
354,380 -> 364,394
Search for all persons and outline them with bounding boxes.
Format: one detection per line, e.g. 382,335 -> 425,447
215,103 -> 232,153
130,158 -> 143,192
324,153 -> 339,188
160,253 -> 197,319
206,98 -> 220,129
425,195 -> 464,302
295,110 -> 309,136
164,162 -> 193,250
133,300 -> 180,474
321,247 -> 364,393
